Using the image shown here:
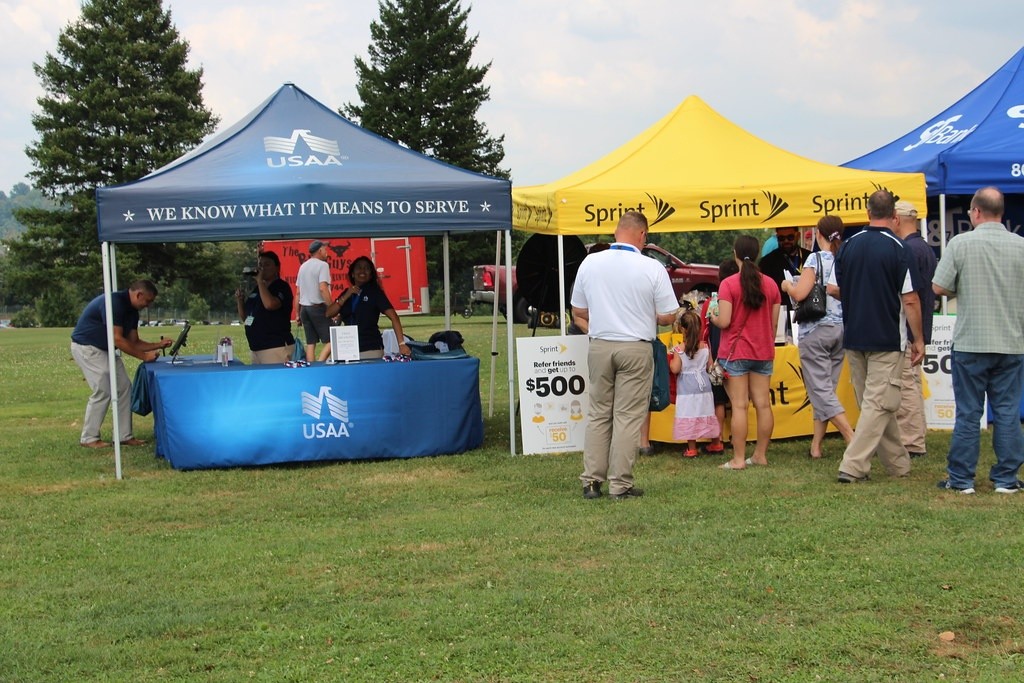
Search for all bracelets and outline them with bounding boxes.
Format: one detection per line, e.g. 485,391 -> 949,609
398,341 -> 406,345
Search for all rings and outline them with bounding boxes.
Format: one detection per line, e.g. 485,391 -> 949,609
353,286 -> 354,289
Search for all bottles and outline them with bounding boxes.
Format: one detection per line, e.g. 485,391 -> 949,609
222,343 -> 228,366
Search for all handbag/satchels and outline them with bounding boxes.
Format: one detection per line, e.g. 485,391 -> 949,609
649,336 -> 670,412
292,327 -> 306,362
708,359 -> 730,385
792,252 -> 827,325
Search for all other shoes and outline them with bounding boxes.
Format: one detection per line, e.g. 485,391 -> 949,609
120,437 -> 149,446
583,481 -> 603,499
909,452 -> 924,459
639,443 -> 656,456
837,471 -> 857,484
682,448 -> 699,459
701,443 -> 724,455
80,440 -> 112,448
609,487 -> 643,499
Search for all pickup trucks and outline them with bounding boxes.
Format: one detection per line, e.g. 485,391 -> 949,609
472,243 -> 721,327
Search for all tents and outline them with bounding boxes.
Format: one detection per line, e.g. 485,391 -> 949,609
90,46 -> 1024,479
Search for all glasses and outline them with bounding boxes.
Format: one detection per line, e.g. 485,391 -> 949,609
968,207 -> 980,216
777,234 -> 794,241
641,231 -> 647,246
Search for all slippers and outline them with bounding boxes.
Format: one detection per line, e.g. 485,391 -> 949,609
718,462 -> 744,470
746,458 -> 753,464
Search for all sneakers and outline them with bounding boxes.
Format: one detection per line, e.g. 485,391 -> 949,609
936,478 -> 975,495
994,479 -> 1024,494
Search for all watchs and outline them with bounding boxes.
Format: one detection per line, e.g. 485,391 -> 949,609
335,299 -> 342,308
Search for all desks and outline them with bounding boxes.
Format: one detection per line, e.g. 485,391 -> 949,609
131,353 -> 483,471
648,344 -> 929,443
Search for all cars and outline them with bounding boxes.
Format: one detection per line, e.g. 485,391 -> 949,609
137,318 -> 244,325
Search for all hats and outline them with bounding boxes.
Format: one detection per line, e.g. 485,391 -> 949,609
895,201 -> 918,216
309,240 -> 328,254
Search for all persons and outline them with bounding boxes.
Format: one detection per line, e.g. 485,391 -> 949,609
931,185 -> 1024,497
326,255 -> 412,360
295,239 -> 339,363
758,189 -> 938,484
69,278 -> 173,448
233,250 -> 296,365
566,211 -> 782,500
